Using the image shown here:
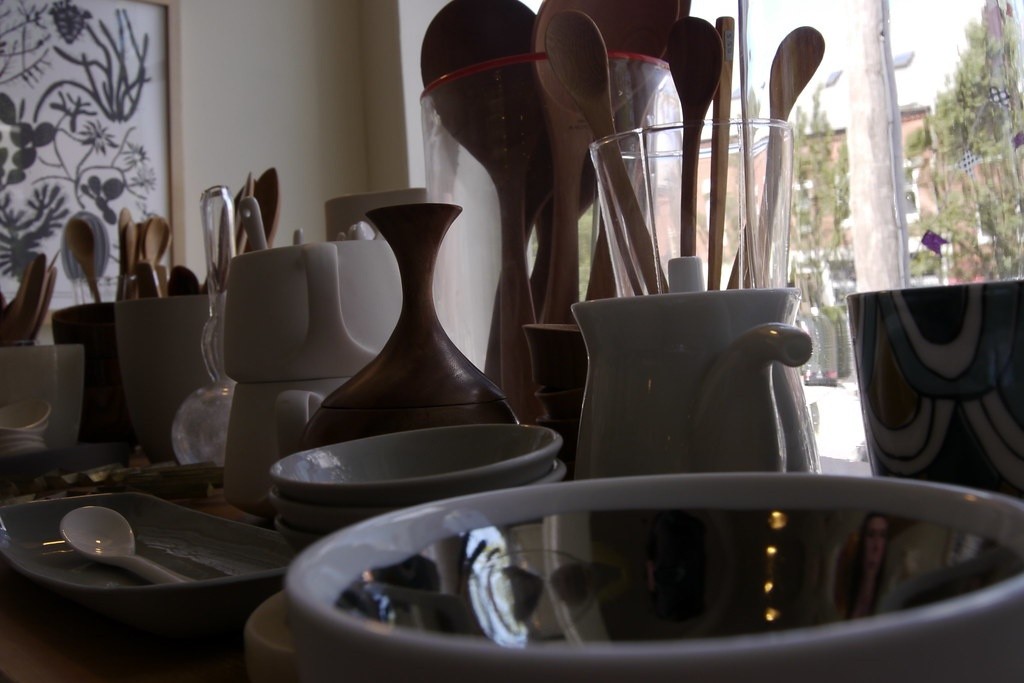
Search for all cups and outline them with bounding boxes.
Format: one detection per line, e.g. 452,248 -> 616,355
221,377 -> 350,518
281,470 -> 1024,683
0,344 -> 85,449
245,591 -> 297,683
52,302 -> 138,450
224,240 -> 403,382
589,118 -> 794,298
116,294 -> 227,463
849,280 -> 1024,505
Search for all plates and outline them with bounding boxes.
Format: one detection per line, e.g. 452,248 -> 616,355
0,400 -> 51,456
0,492 -> 325,612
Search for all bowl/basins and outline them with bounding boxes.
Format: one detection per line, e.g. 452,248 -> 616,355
522,323 -> 588,481
264,424 -> 569,554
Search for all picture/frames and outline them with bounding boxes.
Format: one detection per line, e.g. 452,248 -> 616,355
0,0 -> 187,325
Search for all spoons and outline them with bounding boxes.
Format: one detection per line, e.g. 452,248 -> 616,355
546,11 -> 668,297
668,17 -> 725,259
60,505 -> 194,585
758,26 -> 825,271
68,208 -> 169,304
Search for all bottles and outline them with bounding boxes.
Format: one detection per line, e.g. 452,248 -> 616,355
296,203 -> 519,452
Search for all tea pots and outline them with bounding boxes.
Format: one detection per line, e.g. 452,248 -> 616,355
575,257 -> 822,478
